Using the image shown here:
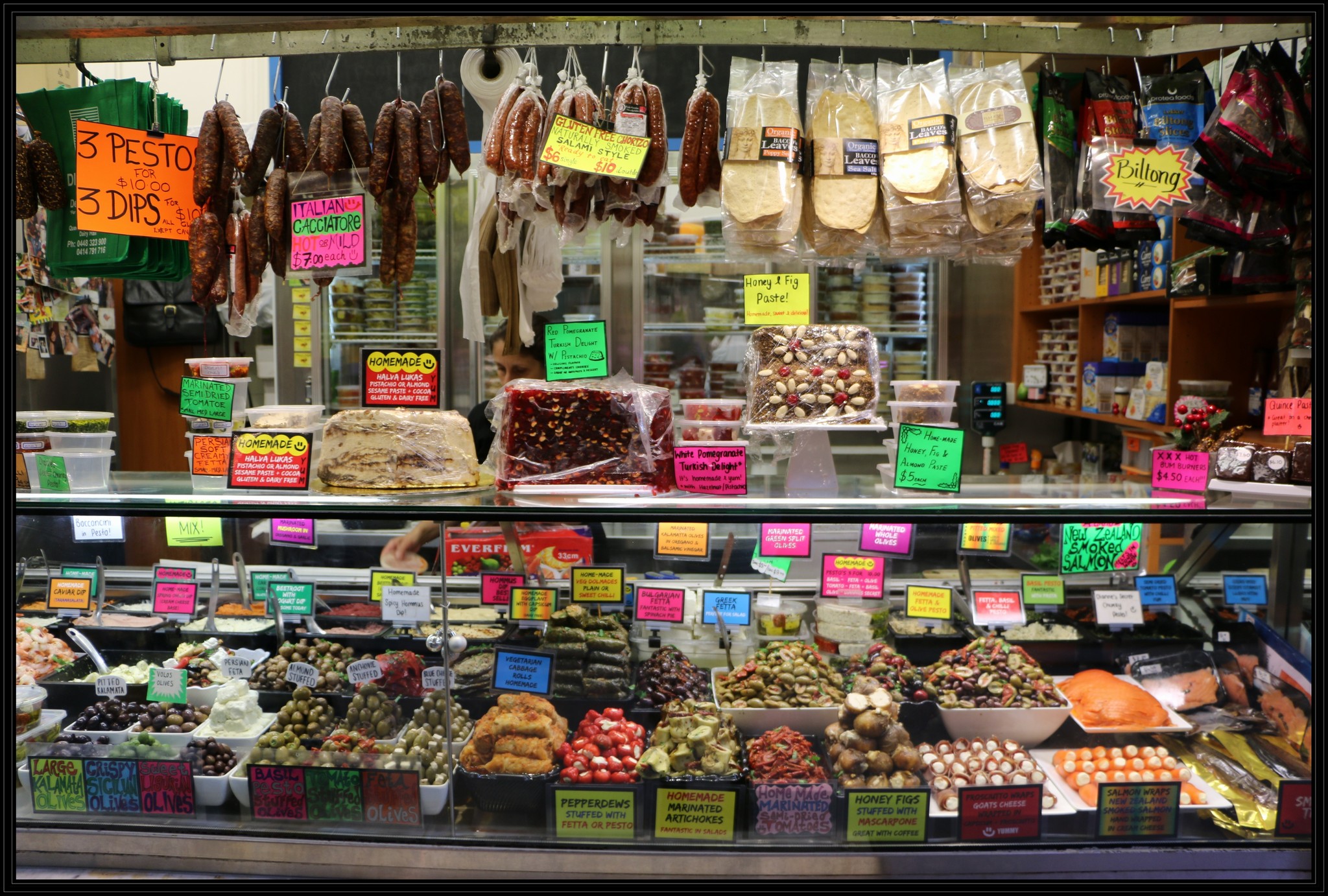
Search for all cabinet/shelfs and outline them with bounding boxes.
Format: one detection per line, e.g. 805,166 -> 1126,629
1012,181 -> 1314,473
639,213 -> 953,416
11,464 -> 1314,886
308,166 -> 478,413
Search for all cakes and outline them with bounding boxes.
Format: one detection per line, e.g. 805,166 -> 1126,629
319,406 -> 478,488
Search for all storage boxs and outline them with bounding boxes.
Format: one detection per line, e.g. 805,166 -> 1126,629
12,453 -> 42,490
9,574 -> 1266,851
181,412 -> 244,433
240,422 -> 327,446
185,449 -> 230,487
185,355 -> 255,376
1027,224 -> 1232,482
13,406 -> 49,433
636,223 -> 964,490
243,400 -> 326,427
47,409 -> 113,433
44,447 -> 115,489
202,377 -> 249,417
305,442 -> 322,479
185,432 -> 234,446
14,431 -> 50,454
44,430 -> 118,451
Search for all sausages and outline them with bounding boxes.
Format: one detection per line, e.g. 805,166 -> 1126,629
14,70 -> 721,332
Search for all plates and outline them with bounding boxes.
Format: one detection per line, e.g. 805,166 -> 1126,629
1029,747 -> 1233,811
1112,622 -> 1311,767
1048,673 -> 1194,732
928,751 -> 1076,817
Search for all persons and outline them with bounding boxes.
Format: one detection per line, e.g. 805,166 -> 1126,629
380,310 -> 608,562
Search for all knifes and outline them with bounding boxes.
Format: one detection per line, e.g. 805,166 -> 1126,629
713,532 -> 734,587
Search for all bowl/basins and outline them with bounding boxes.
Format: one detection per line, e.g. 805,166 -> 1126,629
476,265 -> 600,404
324,181 -> 441,411
638,221 -> 964,499
13,685 -> 476,819
1178,380 -> 1231,396
1024,241 -> 1082,408
182,356 -> 326,490
1201,397 -> 1230,411
14,410 -> 118,491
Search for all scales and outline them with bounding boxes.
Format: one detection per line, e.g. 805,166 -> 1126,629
972,380 -> 1008,475
